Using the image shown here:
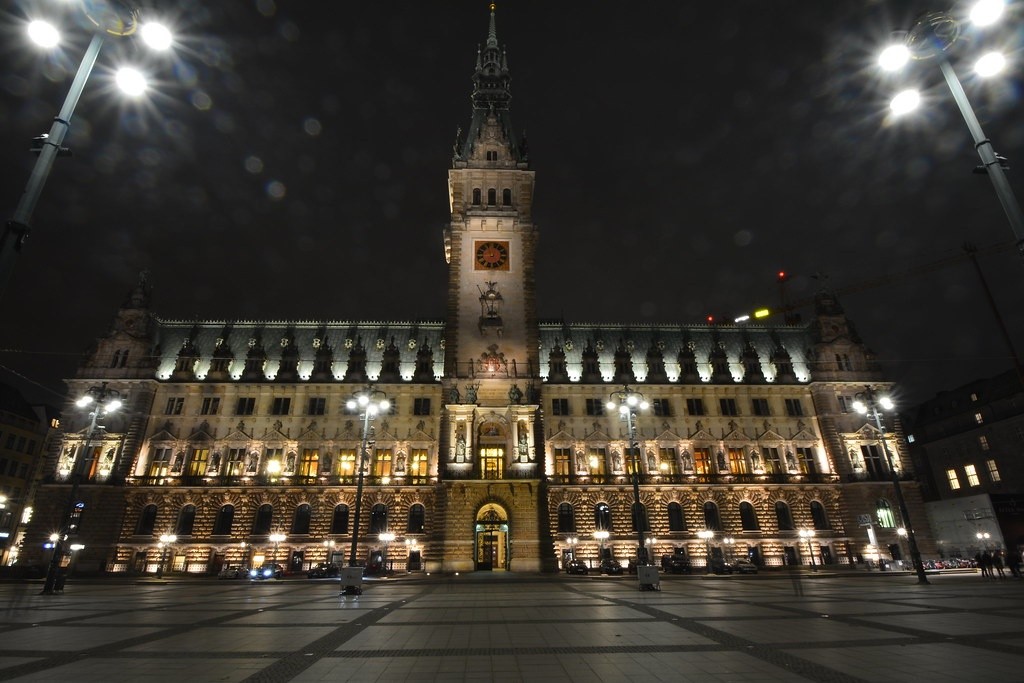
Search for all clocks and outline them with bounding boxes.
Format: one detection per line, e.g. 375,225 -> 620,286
474,240 -> 509,271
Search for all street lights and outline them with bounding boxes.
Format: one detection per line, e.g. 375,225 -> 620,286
593,530 -> 610,559
323,540 -> 336,568
157,534 -> 177,579
876,1 -> 1024,262
646,538 -> 657,564
566,536 -> 578,560
976,532 -> 990,554
269,533 -> 286,578
799,529 -> 818,572
405,539 -> 417,574
379,533 -> 395,577
698,531 -> 715,575
337,383 -> 391,595
605,384 -> 654,591
0,0 -> 174,286
723,537 -> 735,558
39,380 -> 124,596
850,384 -> 931,585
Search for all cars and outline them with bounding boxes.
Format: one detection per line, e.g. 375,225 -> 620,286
706,557 -> 732,574
732,558 -> 757,574
217,566 -> 249,579
566,560 -> 588,574
661,554 -> 692,575
599,558 -> 623,575
306,563 -> 344,579
250,563 -> 283,579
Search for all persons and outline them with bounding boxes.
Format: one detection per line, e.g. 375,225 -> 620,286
993,551 -> 1005,578
982,550 -> 994,576
1006,552 -> 1022,577
975,554 -> 988,577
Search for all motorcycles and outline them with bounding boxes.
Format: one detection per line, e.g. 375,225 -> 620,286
901,558 -> 978,571
